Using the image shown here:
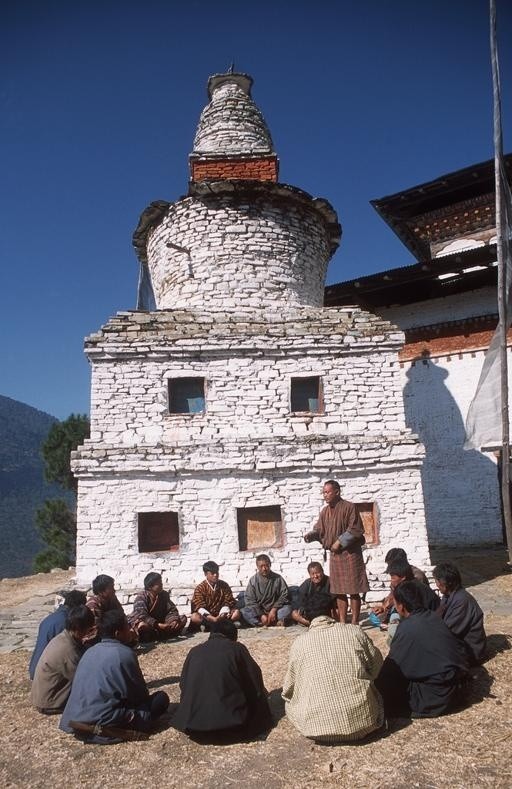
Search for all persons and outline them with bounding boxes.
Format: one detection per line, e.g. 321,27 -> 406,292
191,561 -> 240,631
30,604 -> 94,714
171,617 -> 283,745
303,480 -> 366,624
369,547 -> 441,648
378,579 -> 485,719
434,564 -> 485,666
82,575 -> 136,653
281,592 -> 384,742
133,572 -> 187,652
58,609 -> 169,744
292,562 -> 338,626
240,554 -> 292,627
29,591 -> 87,680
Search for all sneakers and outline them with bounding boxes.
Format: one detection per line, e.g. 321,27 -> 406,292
234,621 -> 241,628
200,624 -> 208,632
282,617 -> 291,626
